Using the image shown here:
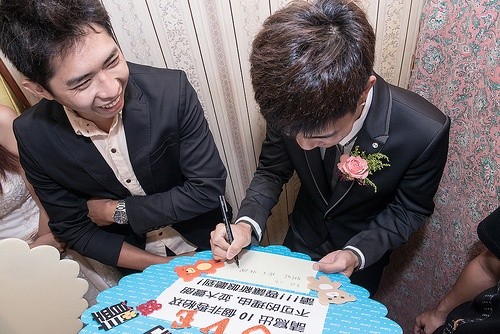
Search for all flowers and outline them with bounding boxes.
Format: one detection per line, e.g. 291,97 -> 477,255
337,145 -> 391,193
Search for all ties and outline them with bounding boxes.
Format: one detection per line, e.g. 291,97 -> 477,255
323,131 -> 359,193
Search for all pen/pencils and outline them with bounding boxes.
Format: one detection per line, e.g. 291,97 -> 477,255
219,195 -> 239,269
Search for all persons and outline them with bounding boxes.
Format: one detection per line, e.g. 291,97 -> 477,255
414,208 -> 500,334
0,0 -> 230,283
210,1 -> 451,301
0,105 -> 64,252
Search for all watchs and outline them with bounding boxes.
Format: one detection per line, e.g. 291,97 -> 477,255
113,199 -> 128,225
